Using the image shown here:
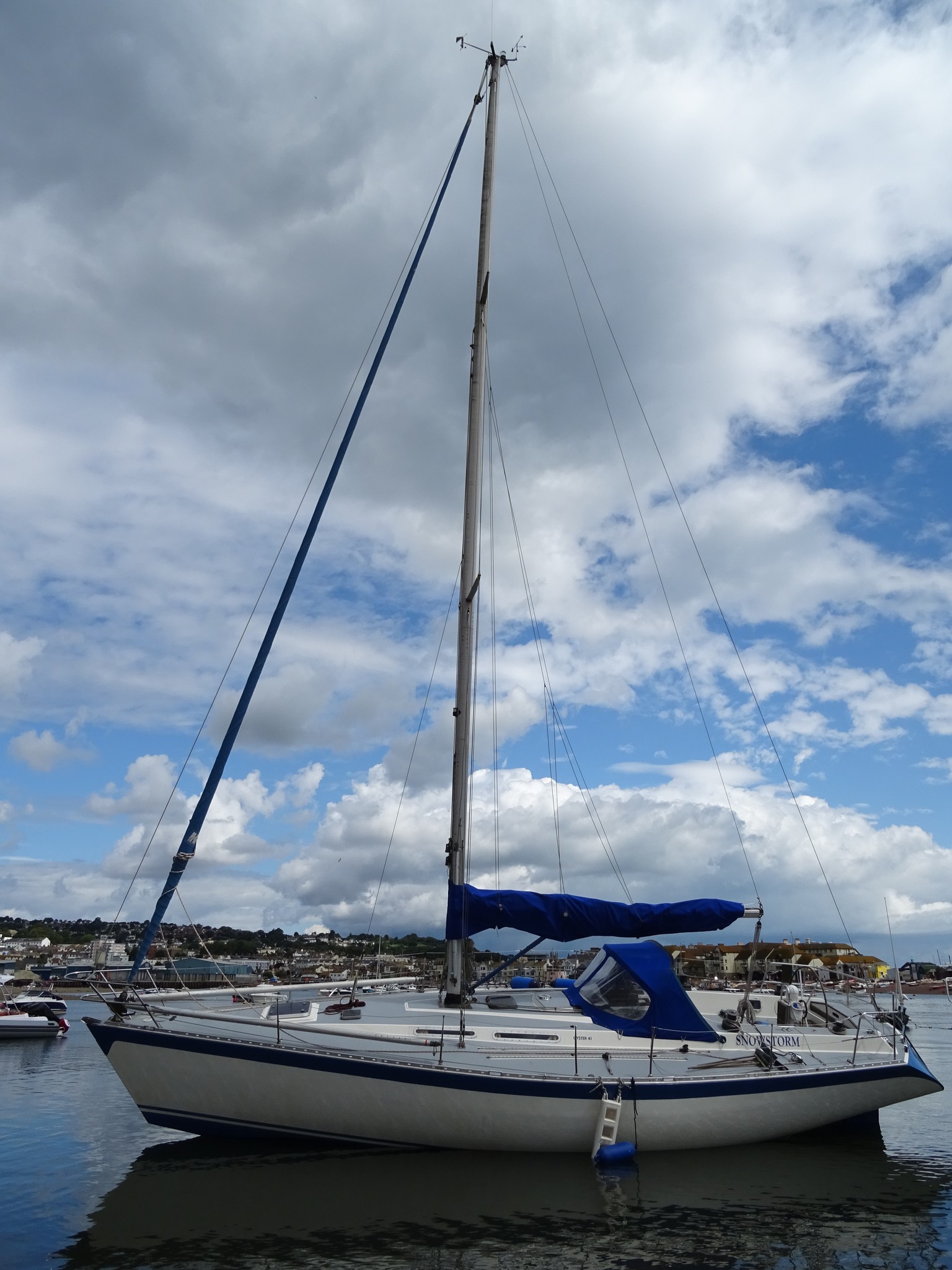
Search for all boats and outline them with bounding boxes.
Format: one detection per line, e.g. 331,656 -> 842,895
319,979 -> 359,996
361,934 -> 417,993
875,976 -> 952,990
849,989 -> 874,997
0,973 -> 71,1039
232,983 -> 288,1005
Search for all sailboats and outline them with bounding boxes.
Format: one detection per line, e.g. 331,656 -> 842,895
74,35 -> 947,1165
